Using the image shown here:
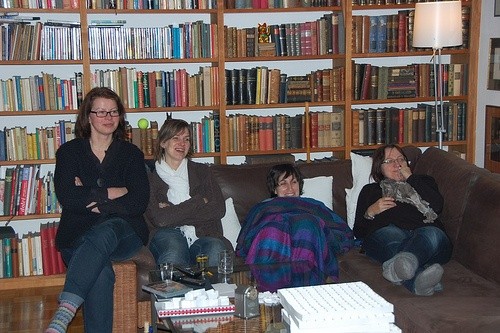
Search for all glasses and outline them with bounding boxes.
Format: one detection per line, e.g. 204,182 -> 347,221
90,109 -> 121,118
381,156 -> 407,166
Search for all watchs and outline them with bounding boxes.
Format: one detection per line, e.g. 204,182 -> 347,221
365,211 -> 374,218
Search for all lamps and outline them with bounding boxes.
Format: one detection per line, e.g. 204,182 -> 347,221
413,2 -> 463,151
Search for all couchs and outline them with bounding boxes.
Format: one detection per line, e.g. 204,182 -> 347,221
112,150 -> 500,333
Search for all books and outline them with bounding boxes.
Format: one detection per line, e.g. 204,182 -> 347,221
125,112 -> 220,154
351,59 -> 468,100
224,66 -> 345,105
88,19 -> 219,60
352,102 -> 467,145
142,279 -> 192,298
225,107 -> 345,151
1,0 -> 461,9
223,10 -> 345,58
0,13 -> 84,280
277,281 -> 402,333
352,7 -> 470,53
90,66 -> 219,109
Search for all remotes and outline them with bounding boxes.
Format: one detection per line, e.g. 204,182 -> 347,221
174,266 -> 207,286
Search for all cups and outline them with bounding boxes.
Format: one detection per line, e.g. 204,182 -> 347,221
160,264 -> 173,282
218,250 -> 233,273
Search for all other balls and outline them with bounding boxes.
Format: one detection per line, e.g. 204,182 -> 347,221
138,119 -> 149,130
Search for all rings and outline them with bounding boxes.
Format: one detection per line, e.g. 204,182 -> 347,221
399,166 -> 402,170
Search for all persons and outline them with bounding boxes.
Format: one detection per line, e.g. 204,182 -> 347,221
142,119 -> 234,267
43,87 -> 150,333
352,144 -> 452,296
265,163 -> 304,198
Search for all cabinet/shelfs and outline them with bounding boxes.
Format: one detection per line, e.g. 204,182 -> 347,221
0,0 -> 482,221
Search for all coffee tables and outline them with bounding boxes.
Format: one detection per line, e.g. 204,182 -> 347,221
151,260 -> 336,333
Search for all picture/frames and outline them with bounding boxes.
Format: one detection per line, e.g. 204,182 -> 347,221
484,104 -> 500,176
486,38 -> 500,92
